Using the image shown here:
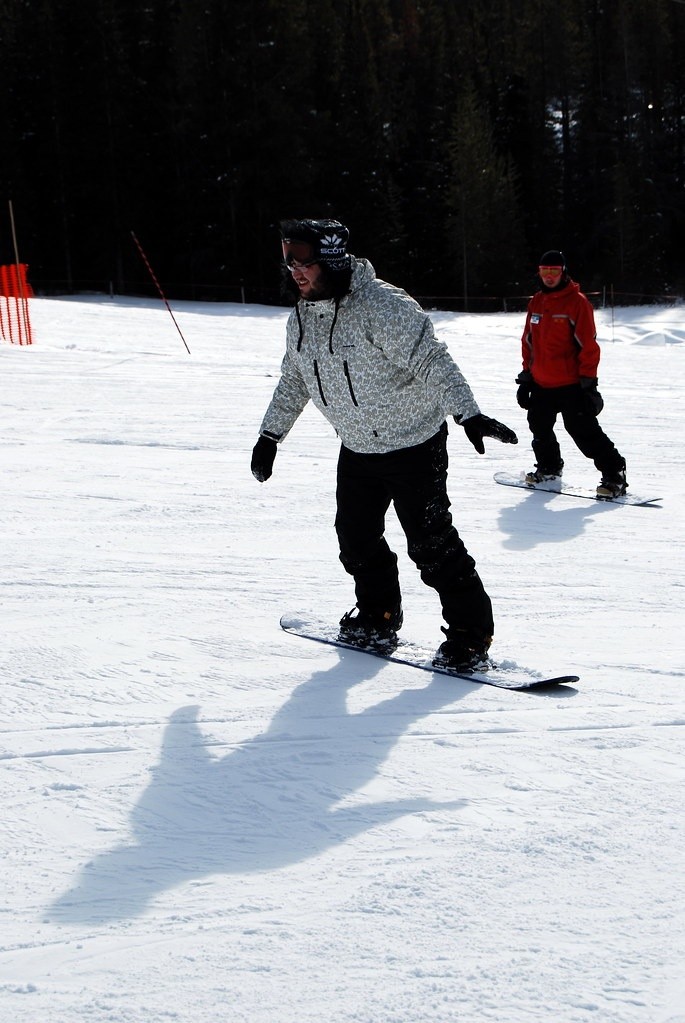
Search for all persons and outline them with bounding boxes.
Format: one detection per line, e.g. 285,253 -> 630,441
515,248 -> 629,499
250,219 -> 518,671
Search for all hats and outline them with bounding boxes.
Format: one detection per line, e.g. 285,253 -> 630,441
538,251 -> 565,268
265,219 -> 351,301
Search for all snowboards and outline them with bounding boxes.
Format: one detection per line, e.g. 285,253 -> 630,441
493,471 -> 664,505
279,609 -> 581,691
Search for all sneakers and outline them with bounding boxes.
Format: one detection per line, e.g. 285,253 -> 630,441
431,638 -> 492,672
338,603 -> 403,646
526,466 -> 563,486
597,469 -> 629,498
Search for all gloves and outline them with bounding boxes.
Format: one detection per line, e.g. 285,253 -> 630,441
579,376 -> 604,417
461,414 -> 519,454
515,370 -> 532,410
251,435 -> 278,483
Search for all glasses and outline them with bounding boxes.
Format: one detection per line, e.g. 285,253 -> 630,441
279,243 -> 318,271
540,267 -> 562,277
